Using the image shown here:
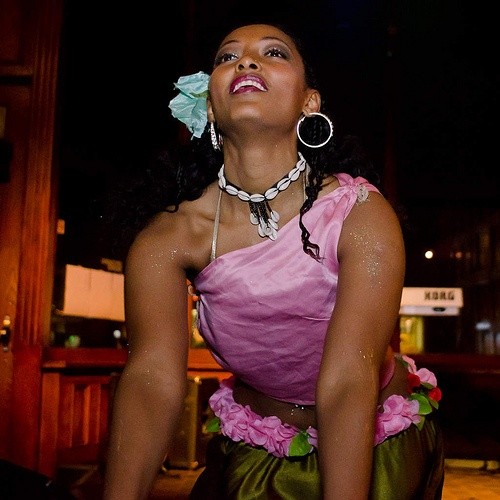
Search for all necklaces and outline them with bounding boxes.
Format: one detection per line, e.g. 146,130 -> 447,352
217,149 -> 309,241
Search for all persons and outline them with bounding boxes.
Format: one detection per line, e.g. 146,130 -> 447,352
99,17 -> 447,499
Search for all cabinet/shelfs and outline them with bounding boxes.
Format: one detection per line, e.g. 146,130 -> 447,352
58,374 -> 110,464
